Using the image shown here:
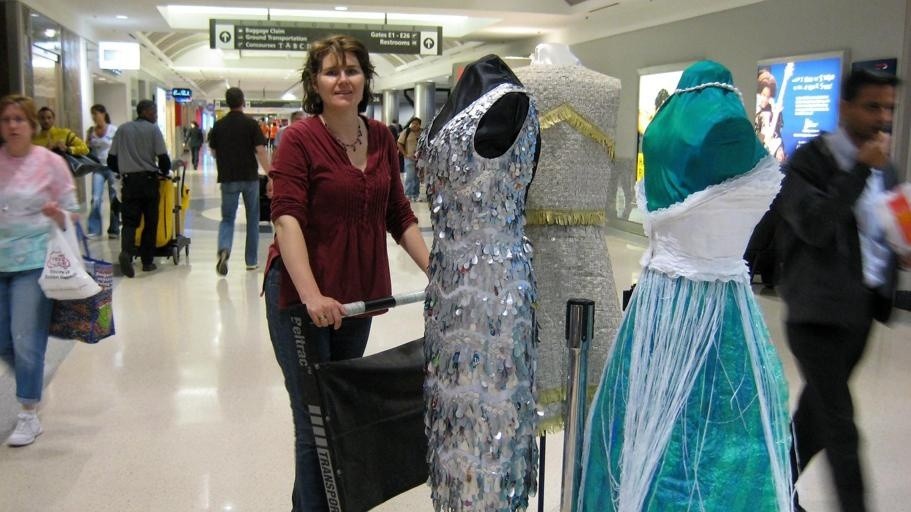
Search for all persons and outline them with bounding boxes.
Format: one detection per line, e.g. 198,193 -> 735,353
33,106 -> 89,158
259,111 -> 304,150
182,120 -> 203,170
86,104 -> 120,240
770,63 -> 905,512
423,53 -> 544,512
512,42 -> 623,434
1,94 -> 82,447
572,60 -> 795,512
107,100 -> 171,279
261,33 -> 429,512
756,71 -> 785,162
209,88 -> 270,275
389,117 -> 423,202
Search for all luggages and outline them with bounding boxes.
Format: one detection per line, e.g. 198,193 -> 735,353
259,171 -> 270,221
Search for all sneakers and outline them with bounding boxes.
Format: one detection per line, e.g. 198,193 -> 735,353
142,263 -> 157,271
215,248 -> 229,275
118,249 -> 135,278
4,408 -> 45,450
245,261 -> 260,270
86,232 -> 103,241
108,232 -> 118,239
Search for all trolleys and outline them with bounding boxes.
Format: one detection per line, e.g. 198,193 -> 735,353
288,280 -> 551,512
129,158 -> 194,267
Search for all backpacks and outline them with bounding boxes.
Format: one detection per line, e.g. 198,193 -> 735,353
741,137 -> 831,301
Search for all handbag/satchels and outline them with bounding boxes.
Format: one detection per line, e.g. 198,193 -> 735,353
63,129 -> 103,178
397,126 -> 411,173
49,216 -> 118,345
